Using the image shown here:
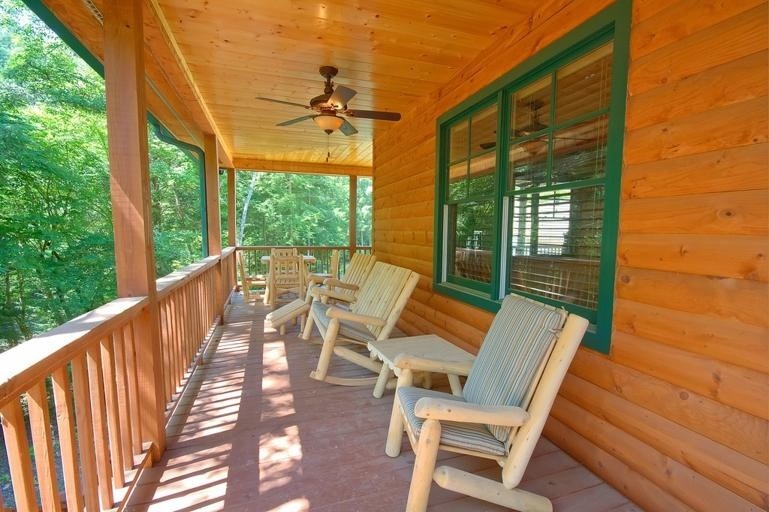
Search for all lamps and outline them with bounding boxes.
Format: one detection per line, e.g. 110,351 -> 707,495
312,115 -> 346,136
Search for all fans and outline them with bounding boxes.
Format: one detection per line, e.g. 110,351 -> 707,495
252,65 -> 402,136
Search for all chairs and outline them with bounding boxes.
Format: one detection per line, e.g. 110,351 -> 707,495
384,291 -> 591,512
307,248 -> 340,302
264,251 -> 377,338
240,253 -> 267,299
271,247 -> 298,255
269,254 -> 304,308
297,261 -> 422,387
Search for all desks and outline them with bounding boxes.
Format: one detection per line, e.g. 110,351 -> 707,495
366,333 -> 477,415
260,255 -> 317,303
